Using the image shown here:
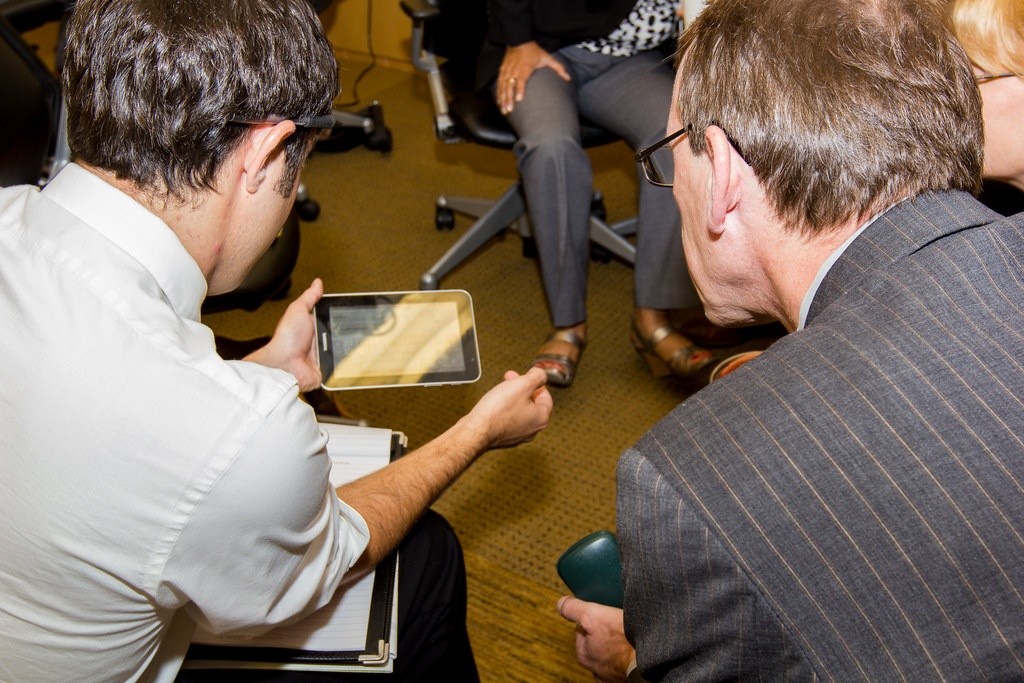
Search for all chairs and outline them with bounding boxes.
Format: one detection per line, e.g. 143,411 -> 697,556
399,0 -> 640,291
1,2 -> 392,222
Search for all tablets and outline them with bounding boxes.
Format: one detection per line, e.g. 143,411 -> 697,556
313,288 -> 481,390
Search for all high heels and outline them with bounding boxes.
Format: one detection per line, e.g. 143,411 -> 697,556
534,326 -> 587,386
631,320 -> 718,377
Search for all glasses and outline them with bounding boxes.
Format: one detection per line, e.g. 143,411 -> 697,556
634,124 -> 692,188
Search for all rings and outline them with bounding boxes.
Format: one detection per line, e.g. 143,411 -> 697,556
506,76 -> 517,84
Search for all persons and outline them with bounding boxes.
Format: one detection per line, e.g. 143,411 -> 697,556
0,0 -> 553,683
431,0 -> 723,388
555,0 -> 1024,683
944,0 -> 1024,205
